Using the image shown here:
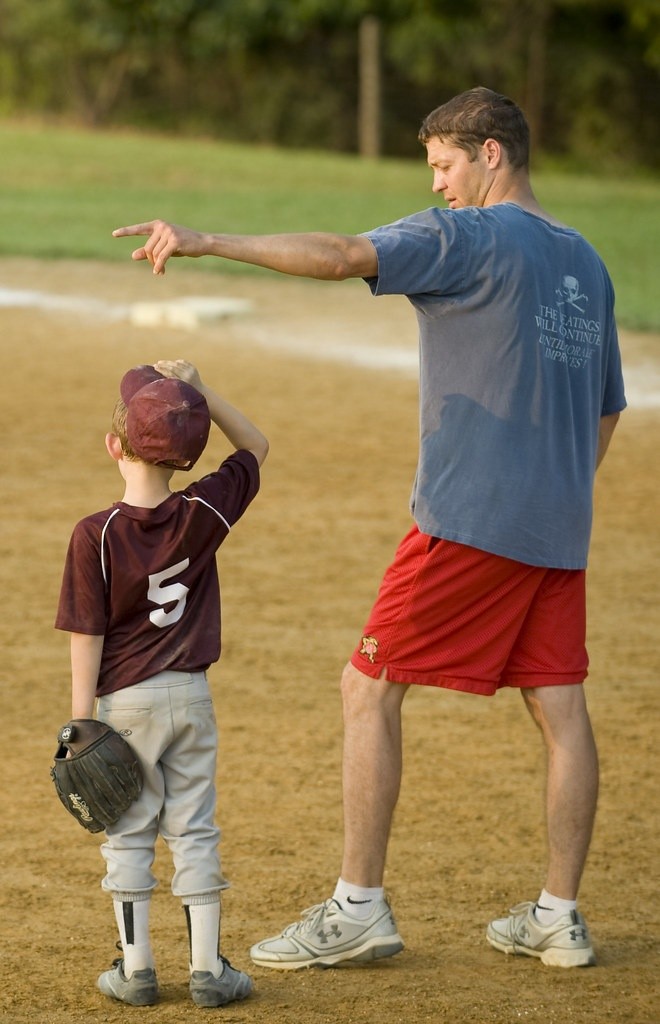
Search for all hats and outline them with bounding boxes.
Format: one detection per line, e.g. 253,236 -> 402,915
119,364 -> 212,471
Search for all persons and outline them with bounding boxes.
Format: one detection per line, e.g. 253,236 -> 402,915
111,82 -> 628,974
52,356 -> 257,1010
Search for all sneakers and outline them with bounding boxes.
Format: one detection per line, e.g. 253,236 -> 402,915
250,898 -> 404,971
189,955 -> 253,1008
98,964 -> 157,1006
488,901 -> 596,968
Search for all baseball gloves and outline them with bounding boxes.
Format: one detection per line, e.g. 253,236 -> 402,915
50,720 -> 143,833
